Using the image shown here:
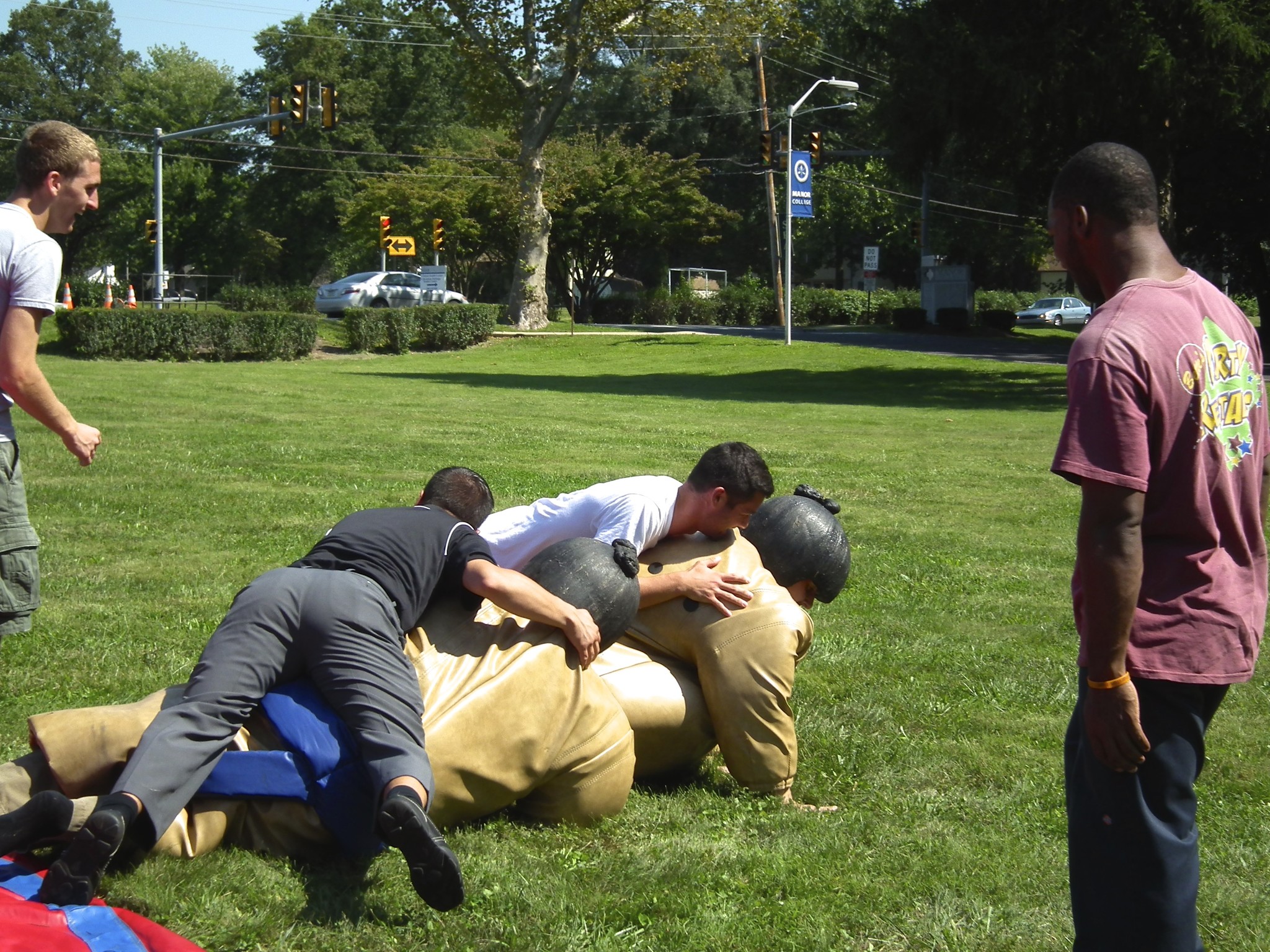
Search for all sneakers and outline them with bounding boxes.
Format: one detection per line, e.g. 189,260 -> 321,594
379,795 -> 463,913
39,808 -> 126,908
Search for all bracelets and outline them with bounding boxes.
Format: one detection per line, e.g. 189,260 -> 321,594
1086,671 -> 1130,689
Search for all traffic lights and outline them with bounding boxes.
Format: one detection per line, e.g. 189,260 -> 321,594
379,215 -> 392,249
288,81 -> 308,125
433,218 -> 446,250
806,131 -> 822,165
758,132 -> 772,169
143,219 -> 159,244
321,83 -> 339,129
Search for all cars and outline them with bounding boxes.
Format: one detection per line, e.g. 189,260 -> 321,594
316,271 -> 471,317
1015,296 -> 1093,329
163,288 -> 199,302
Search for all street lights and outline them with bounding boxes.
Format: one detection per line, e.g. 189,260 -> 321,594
783,78 -> 863,343
760,100 -> 859,327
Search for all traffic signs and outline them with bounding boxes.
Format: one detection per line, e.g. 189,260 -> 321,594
419,266 -> 447,291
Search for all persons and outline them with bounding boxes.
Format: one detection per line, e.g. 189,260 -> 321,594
0,118 -> 126,638
1050,140 -> 1270,952
0,441 -> 853,909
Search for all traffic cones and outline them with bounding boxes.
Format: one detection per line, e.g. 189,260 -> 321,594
61,282 -> 74,310
125,285 -> 139,308
101,284 -> 114,309
